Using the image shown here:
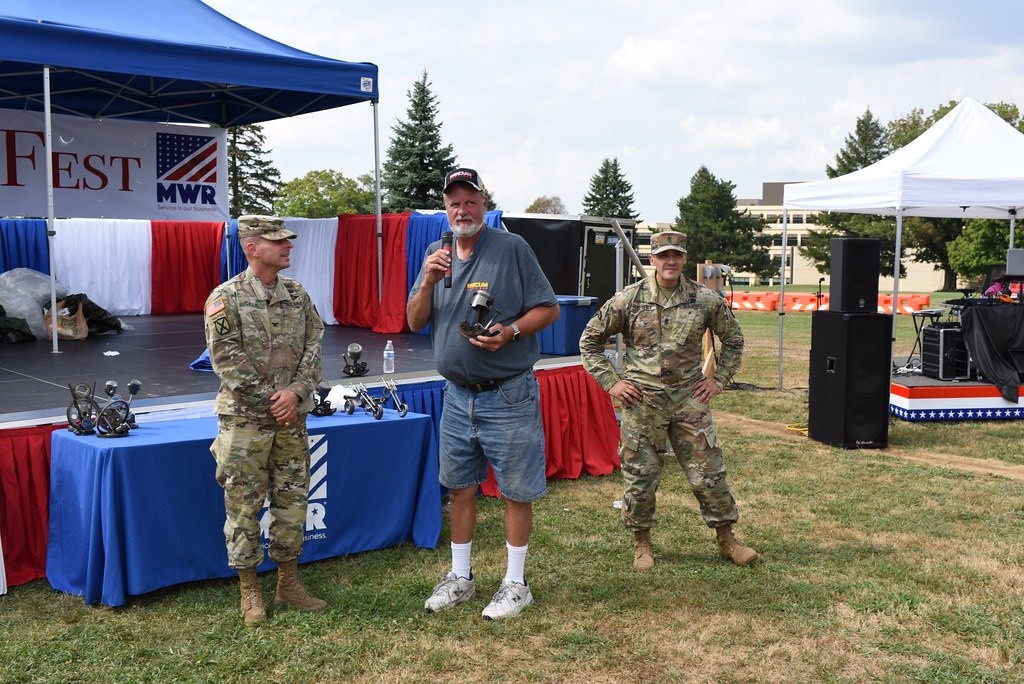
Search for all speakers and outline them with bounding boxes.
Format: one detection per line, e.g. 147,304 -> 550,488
808,310 -> 894,450
828,238 -> 881,314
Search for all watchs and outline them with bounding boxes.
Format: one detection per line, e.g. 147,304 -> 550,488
509,324 -> 521,342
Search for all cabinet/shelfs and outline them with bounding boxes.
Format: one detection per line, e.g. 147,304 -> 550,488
920,325 -> 970,381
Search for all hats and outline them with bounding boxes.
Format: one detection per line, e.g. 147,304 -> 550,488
650,231 -> 689,254
237,215 -> 297,243
443,168 -> 486,193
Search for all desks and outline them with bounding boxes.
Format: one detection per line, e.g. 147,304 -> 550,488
941,302 -> 1024,383
44,406 -> 441,608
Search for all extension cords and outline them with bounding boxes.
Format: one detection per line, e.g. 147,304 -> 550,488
900,367 -> 921,373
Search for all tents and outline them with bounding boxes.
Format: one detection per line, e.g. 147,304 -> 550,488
778,97 -> 1024,390
1,0 -> 385,355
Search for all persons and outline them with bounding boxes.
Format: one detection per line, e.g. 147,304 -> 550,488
407,169 -> 561,621
204,215 -> 329,625
579,231 -> 758,572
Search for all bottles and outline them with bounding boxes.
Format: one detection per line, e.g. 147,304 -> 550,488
383,341 -> 394,373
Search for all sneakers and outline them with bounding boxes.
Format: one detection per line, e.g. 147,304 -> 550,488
482,576 -> 535,620
424,567 -> 476,613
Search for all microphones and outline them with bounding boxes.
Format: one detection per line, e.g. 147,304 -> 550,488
441,230 -> 453,288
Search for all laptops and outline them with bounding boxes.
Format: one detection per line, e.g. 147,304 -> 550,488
991,248 -> 1024,282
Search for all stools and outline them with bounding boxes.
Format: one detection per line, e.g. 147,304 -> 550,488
905,312 -> 943,364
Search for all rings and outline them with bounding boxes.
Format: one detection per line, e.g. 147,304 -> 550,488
285,422 -> 290,426
479,342 -> 486,348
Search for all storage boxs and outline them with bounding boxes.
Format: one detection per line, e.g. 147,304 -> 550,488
536,295 -> 597,355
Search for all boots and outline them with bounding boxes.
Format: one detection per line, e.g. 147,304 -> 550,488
633,529 -> 655,569
275,560 -> 327,610
716,525 -> 759,565
238,567 -> 268,628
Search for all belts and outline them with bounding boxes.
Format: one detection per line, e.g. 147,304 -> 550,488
464,380 -> 500,393
